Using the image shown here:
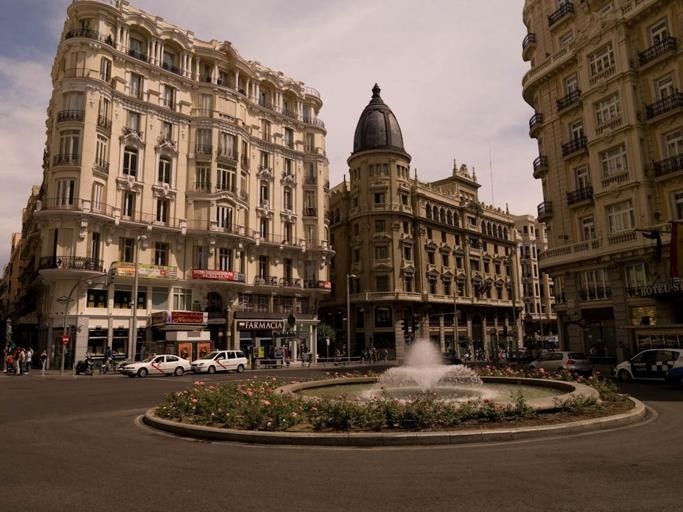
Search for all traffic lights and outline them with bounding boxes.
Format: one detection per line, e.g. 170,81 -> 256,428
412,315 -> 418,331
400,318 -> 408,335
107,272 -> 114,285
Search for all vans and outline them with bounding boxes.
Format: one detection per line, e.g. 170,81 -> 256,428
526,351 -> 593,379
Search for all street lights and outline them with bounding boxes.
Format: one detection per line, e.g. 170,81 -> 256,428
131,235 -> 148,364
346,273 -> 357,364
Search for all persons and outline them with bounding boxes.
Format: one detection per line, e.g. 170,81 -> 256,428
103,346 -> 112,371
40,349 -> 47,370
0,341 -> 33,375
588,339 -> 683,370
334,343 -> 390,363
463,346 -> 485,361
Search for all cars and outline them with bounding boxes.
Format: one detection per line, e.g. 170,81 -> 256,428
115,353 -> 192,378
506,347 -> 530,360
612,348 -> 682,383
191,349 -> 249,374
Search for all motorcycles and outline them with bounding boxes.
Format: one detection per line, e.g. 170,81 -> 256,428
76,352 -> 96,376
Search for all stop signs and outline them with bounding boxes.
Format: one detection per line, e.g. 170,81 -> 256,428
62,336 -> 69,344
